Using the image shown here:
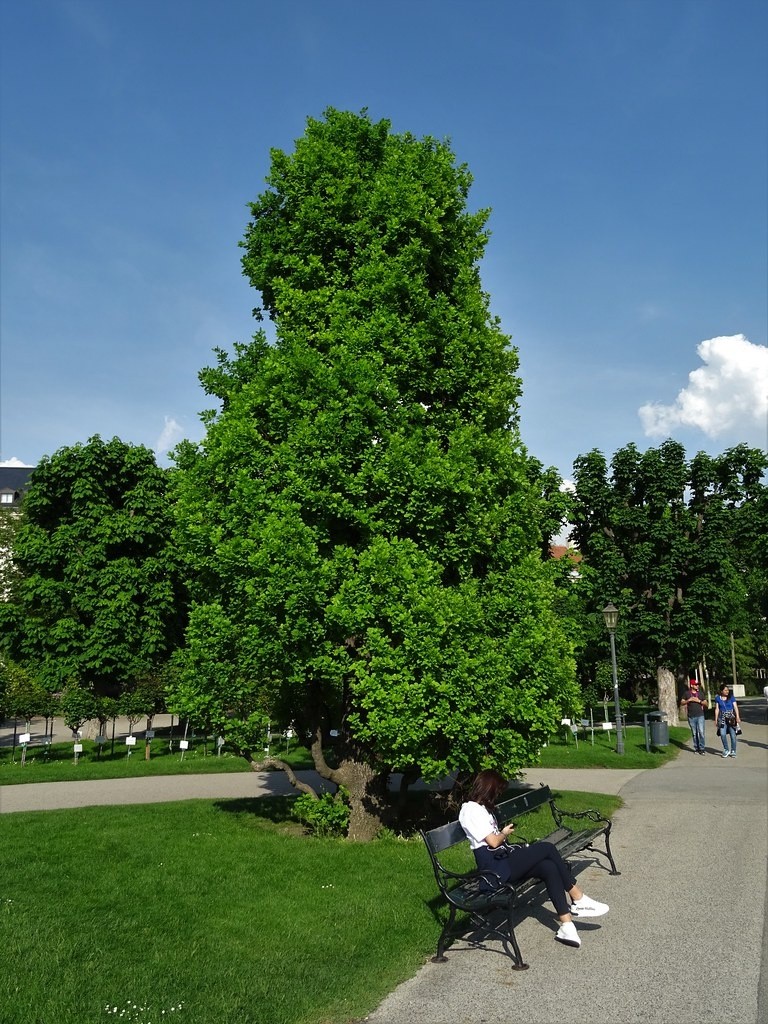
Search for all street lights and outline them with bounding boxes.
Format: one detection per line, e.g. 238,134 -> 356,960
602,599 -> 627,756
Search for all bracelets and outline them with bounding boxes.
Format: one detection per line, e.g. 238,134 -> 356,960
700,700 -> 702,704
500,832 -> 506,837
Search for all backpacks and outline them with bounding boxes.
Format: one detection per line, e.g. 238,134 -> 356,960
690,690 -> 704,710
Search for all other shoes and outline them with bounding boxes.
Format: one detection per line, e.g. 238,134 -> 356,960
695,750 -> 705,755
731,749 -> 736,758
722,749 -> 730,758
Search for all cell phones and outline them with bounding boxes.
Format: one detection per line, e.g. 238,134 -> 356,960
510,823 -> 518,829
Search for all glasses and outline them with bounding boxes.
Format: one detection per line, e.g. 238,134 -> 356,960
695,684 -> 700,686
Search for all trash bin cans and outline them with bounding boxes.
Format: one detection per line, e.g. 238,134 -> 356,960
648,711 -> 670,747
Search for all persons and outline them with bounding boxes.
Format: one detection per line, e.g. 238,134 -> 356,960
681,680 -> 707,756
715,684 -> 741,758
459,769 -> 610,948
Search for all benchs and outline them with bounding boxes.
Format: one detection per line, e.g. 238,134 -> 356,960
419,782 -> 622,971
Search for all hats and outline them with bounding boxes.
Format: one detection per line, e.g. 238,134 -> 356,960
691,680 -> 701,685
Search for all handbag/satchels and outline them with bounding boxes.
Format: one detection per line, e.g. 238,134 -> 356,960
725,717 -> 737,727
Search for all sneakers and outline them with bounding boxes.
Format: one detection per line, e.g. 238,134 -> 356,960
555,921 -> 581,948
570,893 -> 610,918
